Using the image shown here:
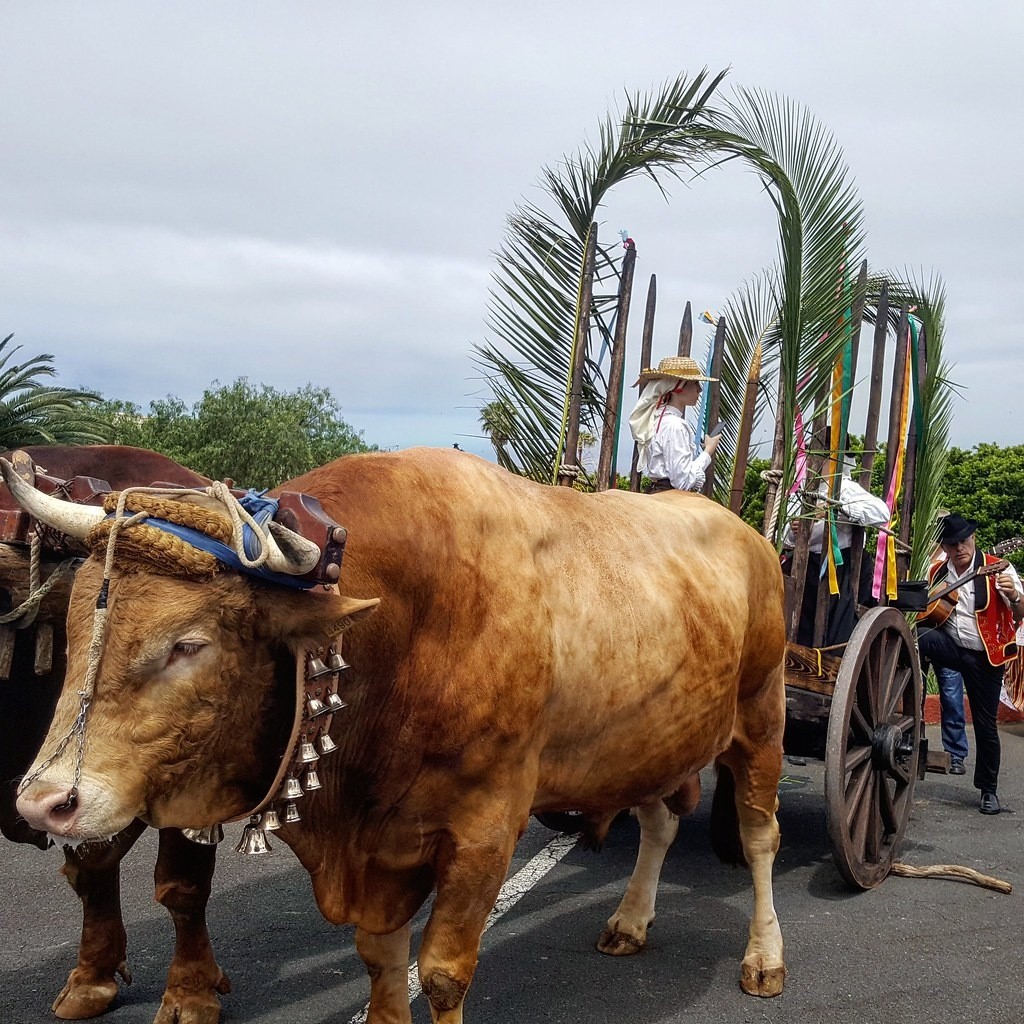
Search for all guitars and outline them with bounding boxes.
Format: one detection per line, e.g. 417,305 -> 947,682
913,559 -> 1010,628
984,537 -> 1024,556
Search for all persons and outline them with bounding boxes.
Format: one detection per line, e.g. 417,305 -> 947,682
918,508 -> 969,774
778,426 -> 891,659
629,357 -> 723,496
912,514 -> 1024,814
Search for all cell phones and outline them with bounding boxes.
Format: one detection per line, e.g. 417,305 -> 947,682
710,421 -> 726,438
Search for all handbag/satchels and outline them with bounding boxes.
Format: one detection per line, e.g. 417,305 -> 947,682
886,580 -> 929,611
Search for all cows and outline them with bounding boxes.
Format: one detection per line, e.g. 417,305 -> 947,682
1,443 -> 788,1024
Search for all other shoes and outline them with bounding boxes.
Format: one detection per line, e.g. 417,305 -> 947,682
978,787 -> 1001,815
950,759 -> 966,774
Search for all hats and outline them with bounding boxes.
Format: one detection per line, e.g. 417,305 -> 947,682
805,426 -> 856,459
638,356 -> 720,382
929,512 -> 978,545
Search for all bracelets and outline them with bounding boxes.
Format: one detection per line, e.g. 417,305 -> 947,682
1009,590 -> 1021,606
